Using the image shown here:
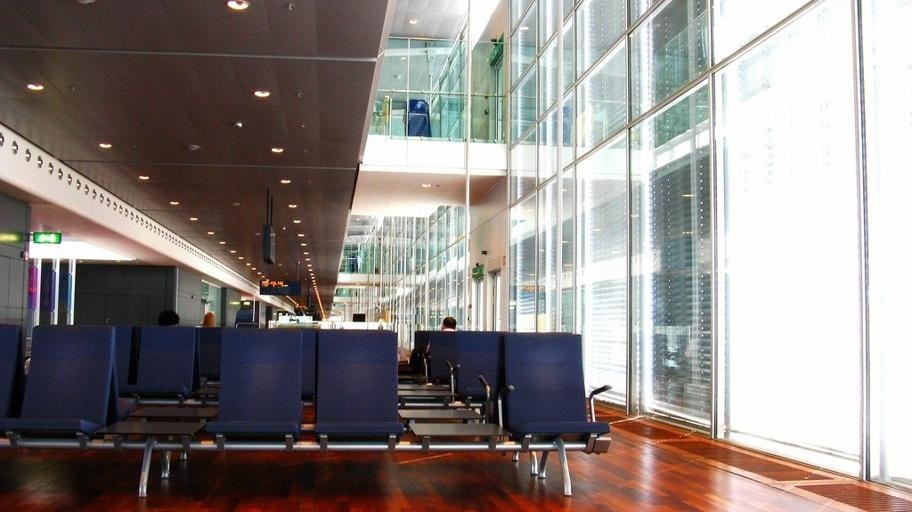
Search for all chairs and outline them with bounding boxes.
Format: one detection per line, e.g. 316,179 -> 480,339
0,324 -> 610,498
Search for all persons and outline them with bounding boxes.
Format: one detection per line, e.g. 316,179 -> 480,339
202,311 -> 216,327
159,310 -> 179,325
442,316 -> 456,331
